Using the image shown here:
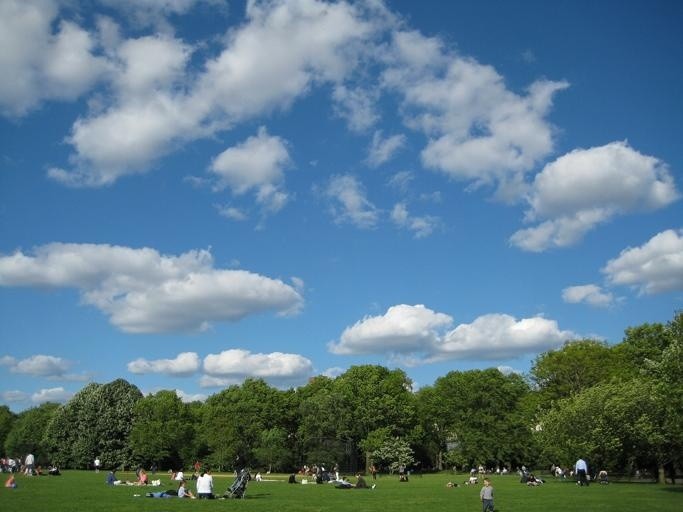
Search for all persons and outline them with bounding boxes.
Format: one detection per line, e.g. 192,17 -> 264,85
0,452 -> 60,488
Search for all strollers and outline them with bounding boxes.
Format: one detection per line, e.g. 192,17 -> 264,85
600,470 -> 608,485
223,470 -> 252,499
